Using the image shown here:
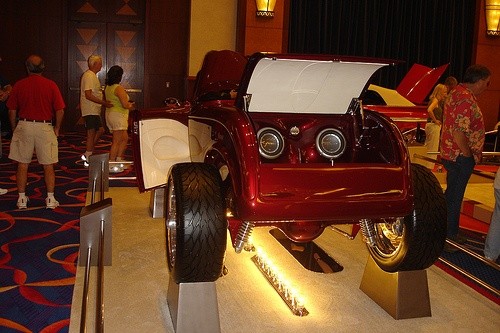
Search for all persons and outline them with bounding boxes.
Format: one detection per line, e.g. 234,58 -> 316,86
440,64 -> 493,245
484,166 -> 500,261
0,58 -> 14,195
424,76 -> 458,171
6,54 -> 66,209
105,64 -> 137,173
80,54 -> 115,166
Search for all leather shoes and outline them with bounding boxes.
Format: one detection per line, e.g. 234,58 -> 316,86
442,242 -> 460,252
453,236 -> 467,244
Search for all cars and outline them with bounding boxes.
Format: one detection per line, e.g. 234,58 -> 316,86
128,49 -> 448,285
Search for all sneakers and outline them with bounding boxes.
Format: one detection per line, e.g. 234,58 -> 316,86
108,160 -> 124,172
17,195 -> 29,209
116,159 -> 131,169
84,160 -> 90,169
80,154 -> 87,161
0,188 -> 9,195
45,196 -> 59,210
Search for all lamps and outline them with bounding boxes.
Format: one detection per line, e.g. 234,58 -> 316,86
485,0 -> 500,37
256,0 -> 277,18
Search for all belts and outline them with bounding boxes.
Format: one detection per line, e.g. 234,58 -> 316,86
427,120 -> 442,124
17,118 -> 53,123
